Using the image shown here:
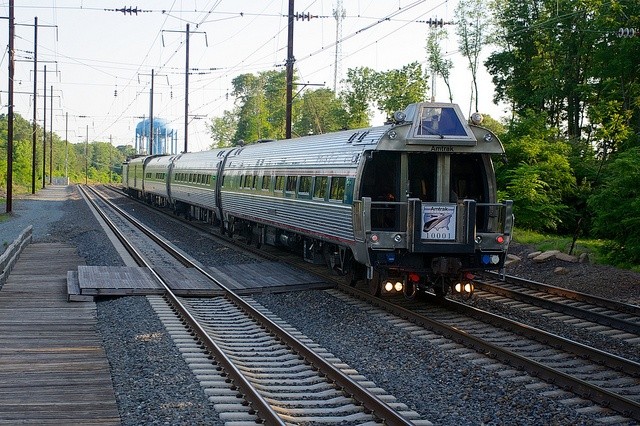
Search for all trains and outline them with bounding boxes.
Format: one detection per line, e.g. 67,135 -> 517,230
122,103 -> 513,301
